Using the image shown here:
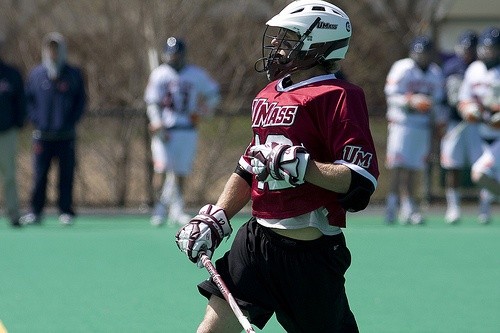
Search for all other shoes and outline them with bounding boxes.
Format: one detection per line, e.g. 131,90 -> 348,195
147,211 -> 194,230
18,211 -> 73,226
382,206 -> 492,227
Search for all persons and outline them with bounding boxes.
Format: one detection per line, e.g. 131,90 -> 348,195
175,0 -> 380,333
24,31 -> 88,225
383,26 -> 500,224
0,58 -> 29,225
142,36 -> 219,226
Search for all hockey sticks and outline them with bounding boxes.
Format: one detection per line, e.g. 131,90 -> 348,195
200,252 -> 255,333
144,17 -> 183,227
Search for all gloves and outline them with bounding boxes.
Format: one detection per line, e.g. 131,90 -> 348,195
248,142 -> 310,187
175,204 -> 234,269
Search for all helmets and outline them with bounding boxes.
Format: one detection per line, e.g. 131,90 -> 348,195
253,0 -> 353,82
161,36 -> 187,54
408,25 -> 500,51
